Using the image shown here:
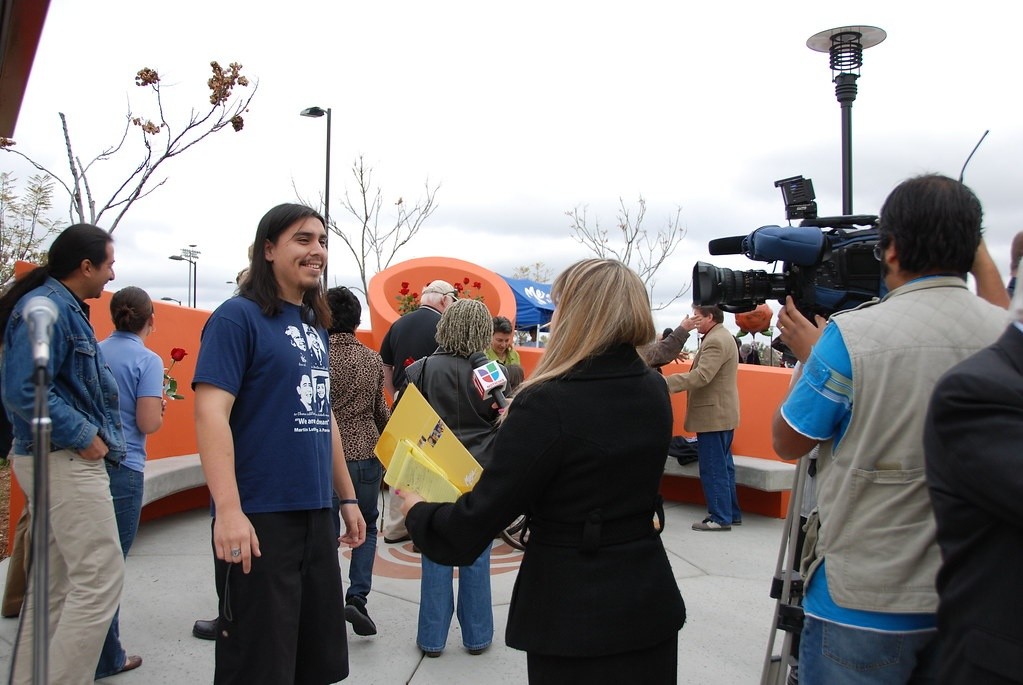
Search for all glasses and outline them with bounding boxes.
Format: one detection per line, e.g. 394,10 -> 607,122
443,288 -> 458,297
872,241 -> 886,261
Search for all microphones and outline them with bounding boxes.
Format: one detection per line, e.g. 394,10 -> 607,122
708,235 -> 748,255
22,296 -> 58,370
469,352 -> 509,409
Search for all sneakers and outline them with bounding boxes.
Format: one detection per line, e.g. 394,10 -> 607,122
692,517 -> 731,531
702,514 -> 742,525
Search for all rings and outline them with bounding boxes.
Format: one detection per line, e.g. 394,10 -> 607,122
779,326 -> 784,332
231,549 -> 241,557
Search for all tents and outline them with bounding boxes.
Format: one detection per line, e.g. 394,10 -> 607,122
498,276 -> 556,348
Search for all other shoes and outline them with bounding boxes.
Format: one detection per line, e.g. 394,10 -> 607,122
384,534 -> 421,552
342,596 -> 377,636
122,654 -> 142,670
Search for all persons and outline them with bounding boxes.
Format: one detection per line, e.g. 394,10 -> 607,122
191,204 -> 367,685
1,225 -> 761,685
923,257 -> 1023,685
771,176 -> 1014,685
1007,231 -> 1023,299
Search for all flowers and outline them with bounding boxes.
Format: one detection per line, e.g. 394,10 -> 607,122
396,277 -> 484,317
163,347 -> 188,401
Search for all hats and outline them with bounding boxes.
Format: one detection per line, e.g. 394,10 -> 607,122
422,279 -> 460,302
740,344 -> 752,356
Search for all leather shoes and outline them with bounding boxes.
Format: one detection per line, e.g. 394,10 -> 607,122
193,616 -> 219,640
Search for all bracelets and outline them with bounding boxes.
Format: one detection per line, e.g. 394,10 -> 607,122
340,499 -> 358,505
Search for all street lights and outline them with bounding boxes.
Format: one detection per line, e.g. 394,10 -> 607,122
169,255 -> 196,308
180,244 -> 201,307
805,24 -> 886,216
161,296 -> 181,306
299,105 -> 332,293
